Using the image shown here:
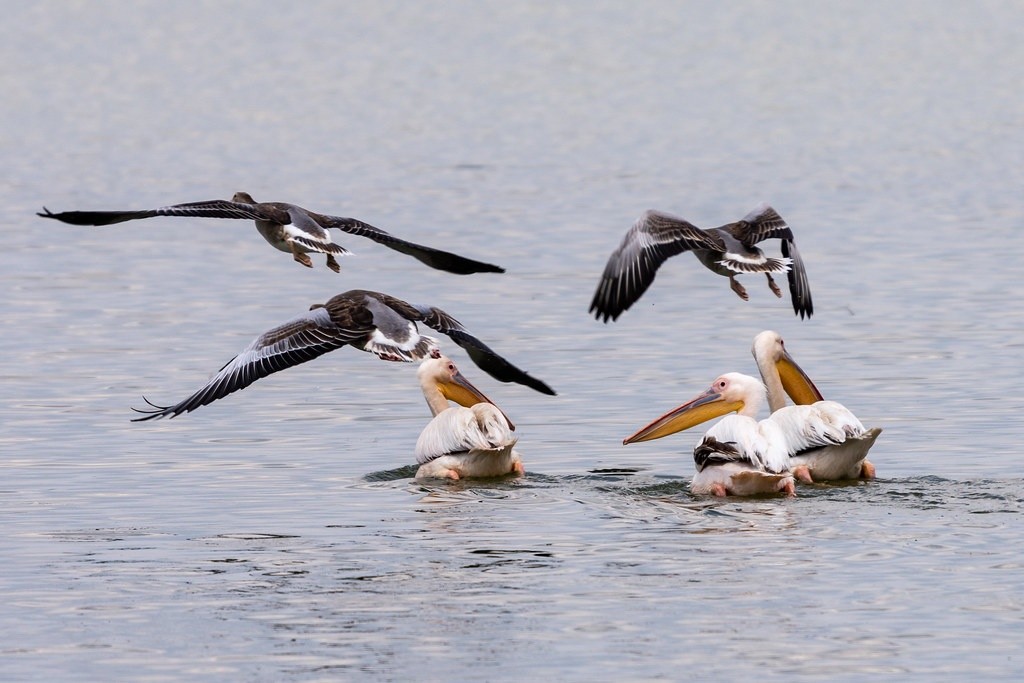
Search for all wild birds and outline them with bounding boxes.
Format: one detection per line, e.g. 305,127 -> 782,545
129,288 -> 557,424
622,372 -> 796,498
415,353 -> 525,478
587,203 -> 815,324
750,329 -> 883,483
35,191 -> 507,277
